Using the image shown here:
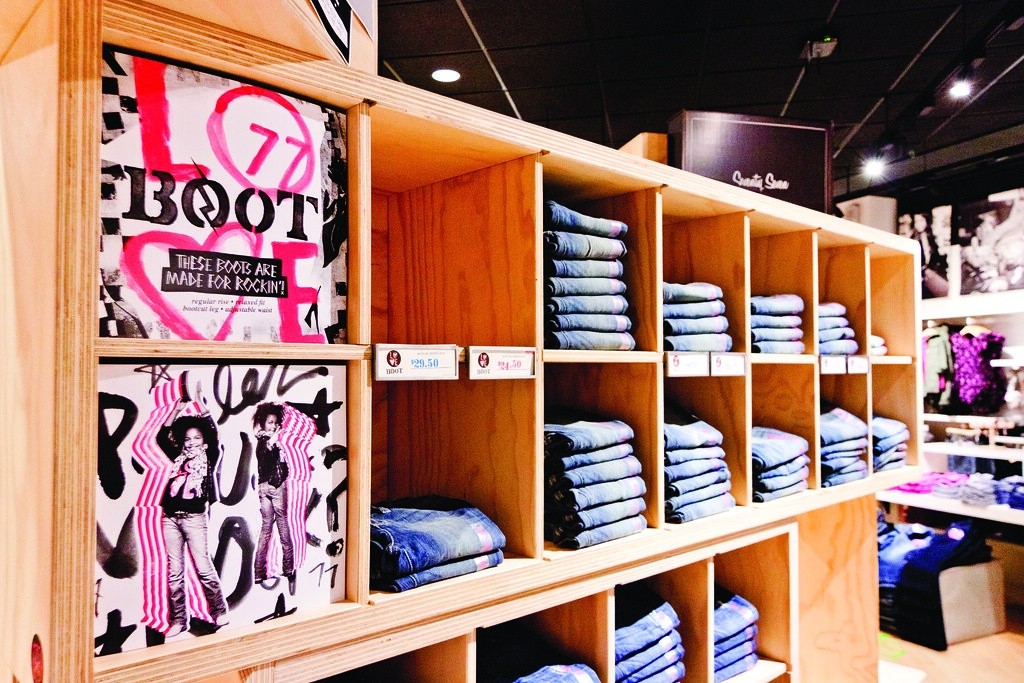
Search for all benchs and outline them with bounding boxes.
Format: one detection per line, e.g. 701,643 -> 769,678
937,557 -> 1007,648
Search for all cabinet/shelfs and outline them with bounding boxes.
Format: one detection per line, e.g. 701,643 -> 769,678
876,440 -> 1024,525
0,0 -> 926,683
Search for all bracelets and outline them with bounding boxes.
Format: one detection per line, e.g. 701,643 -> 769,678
201,410 -> 210,416
267,441 -> 275,449
922,266 -> 928,278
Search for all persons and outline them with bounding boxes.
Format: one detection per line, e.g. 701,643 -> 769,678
156,380 -> 230,638
251,402 -> 297,596
908,189 -> 1024,299
